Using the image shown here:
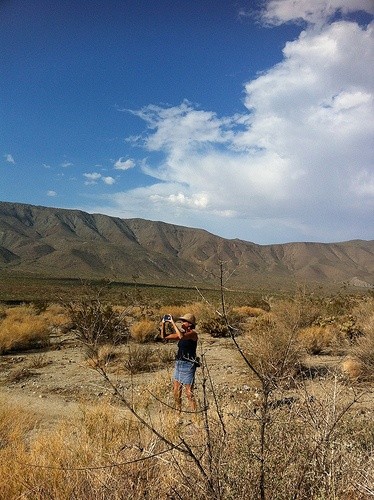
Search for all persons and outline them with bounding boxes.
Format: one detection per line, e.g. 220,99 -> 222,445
161,313 -> 198,429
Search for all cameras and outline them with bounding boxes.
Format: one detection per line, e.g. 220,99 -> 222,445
163,314 -> 170,321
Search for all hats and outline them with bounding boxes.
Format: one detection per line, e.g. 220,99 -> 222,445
177,313 -> 197,326
